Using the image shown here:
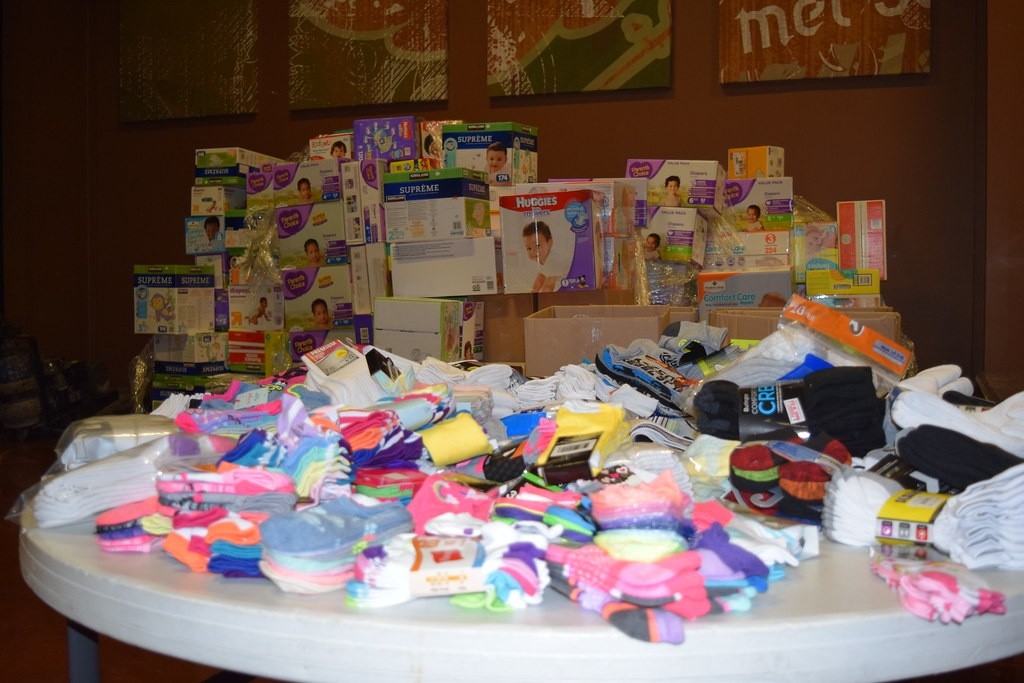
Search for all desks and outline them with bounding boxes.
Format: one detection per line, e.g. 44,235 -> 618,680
15,423 -> 1024,681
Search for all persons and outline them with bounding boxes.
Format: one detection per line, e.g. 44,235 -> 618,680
204,216 -> 220,241
330,141 -> 347,159
298,178 -> 315,205
742,205 -> 766,231
641,233 -> 661,261
484,141 -> 512,186
304,238 -> 324,269
209,200 -> 216,213
249,297 -> 271,325
660,175 -> 685,207
522,221 -> 572,292
465,341 -> 474,360
311,298 -> 334,330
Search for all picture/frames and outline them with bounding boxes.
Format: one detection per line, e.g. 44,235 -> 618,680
486,0 -> 673,102
717,0 -> 933,85
117,1 -> 257,123
287,1 -> 446,115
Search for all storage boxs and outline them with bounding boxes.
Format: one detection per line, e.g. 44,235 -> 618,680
128,114 -> 912,416
